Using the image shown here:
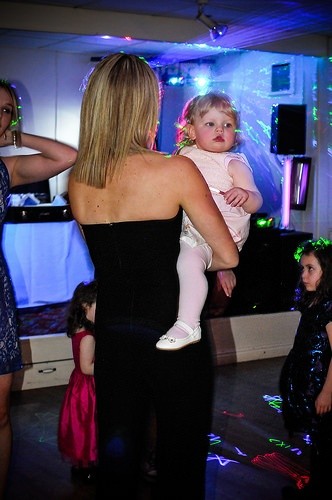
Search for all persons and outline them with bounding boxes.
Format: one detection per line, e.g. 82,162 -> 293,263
279,236 -> 332,500
0,77 -> 79,500
57,279 -> 98,484
156,90 -> 263,350
67,52 -> 240,500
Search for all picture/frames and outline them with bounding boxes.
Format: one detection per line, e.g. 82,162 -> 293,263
268,59 -> 295,95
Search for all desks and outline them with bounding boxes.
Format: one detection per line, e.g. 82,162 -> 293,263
0,220 -> 95,308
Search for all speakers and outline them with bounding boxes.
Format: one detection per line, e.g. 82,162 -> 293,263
269,104 -> 307,155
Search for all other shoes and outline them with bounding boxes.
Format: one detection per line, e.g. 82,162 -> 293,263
155,321 -> 201,350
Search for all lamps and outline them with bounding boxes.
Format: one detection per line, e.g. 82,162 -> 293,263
195,1 -> 228,42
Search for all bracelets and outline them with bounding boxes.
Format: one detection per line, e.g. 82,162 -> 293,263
12,129 -> 18,149
